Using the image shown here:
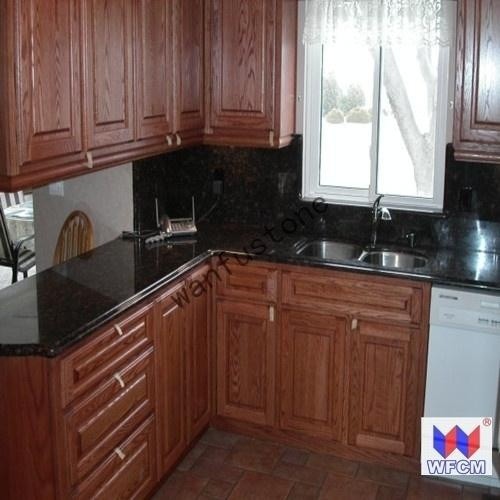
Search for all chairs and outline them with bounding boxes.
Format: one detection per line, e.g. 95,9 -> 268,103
0,190 -> 36,285
53,210 -> 94,267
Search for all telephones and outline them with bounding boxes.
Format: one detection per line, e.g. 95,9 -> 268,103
145,236 -> 198,250
144,196 -> 198,244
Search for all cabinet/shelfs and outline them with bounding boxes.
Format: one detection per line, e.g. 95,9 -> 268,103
210,254 -> 281,442
281,264 -> 432,474
452,0 -> 500,165
0,300 -> 158,499
0,1 -> 136,192
204,1 -> 297,149
158,259 -> 210,488
136,1 -> 204,160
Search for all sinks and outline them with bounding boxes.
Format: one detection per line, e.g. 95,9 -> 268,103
360,246 -> 432,278
289,238 -> 363,265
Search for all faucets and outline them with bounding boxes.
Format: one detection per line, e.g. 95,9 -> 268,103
369,193 -> 392,249
